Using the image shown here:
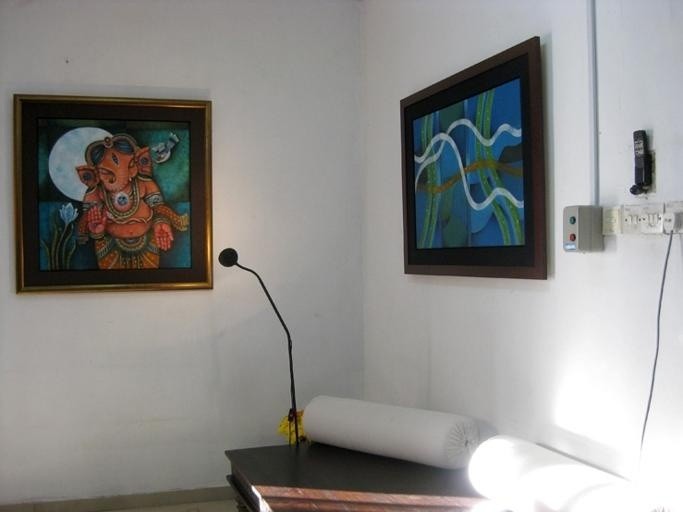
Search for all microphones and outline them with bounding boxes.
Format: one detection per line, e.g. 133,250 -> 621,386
218,248 -> 298,444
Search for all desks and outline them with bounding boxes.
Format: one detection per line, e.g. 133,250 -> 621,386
224,446 -> 489,511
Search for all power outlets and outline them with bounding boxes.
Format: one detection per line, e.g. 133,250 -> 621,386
664,201 -> 683,235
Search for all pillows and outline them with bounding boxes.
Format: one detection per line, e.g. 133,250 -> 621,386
304,396 -> 477,471
465,432 -> 635,510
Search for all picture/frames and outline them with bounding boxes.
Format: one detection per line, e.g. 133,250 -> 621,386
399,35 -> 550,283
10,92 -> 214,293
